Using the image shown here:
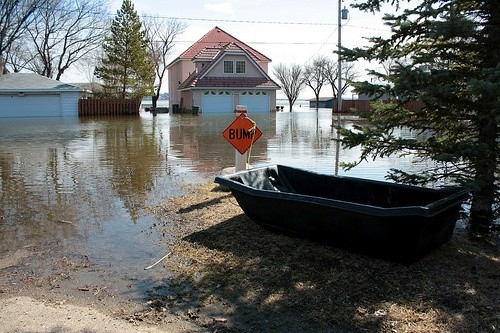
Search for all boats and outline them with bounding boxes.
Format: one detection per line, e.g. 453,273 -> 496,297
213,164 -> 472,265
